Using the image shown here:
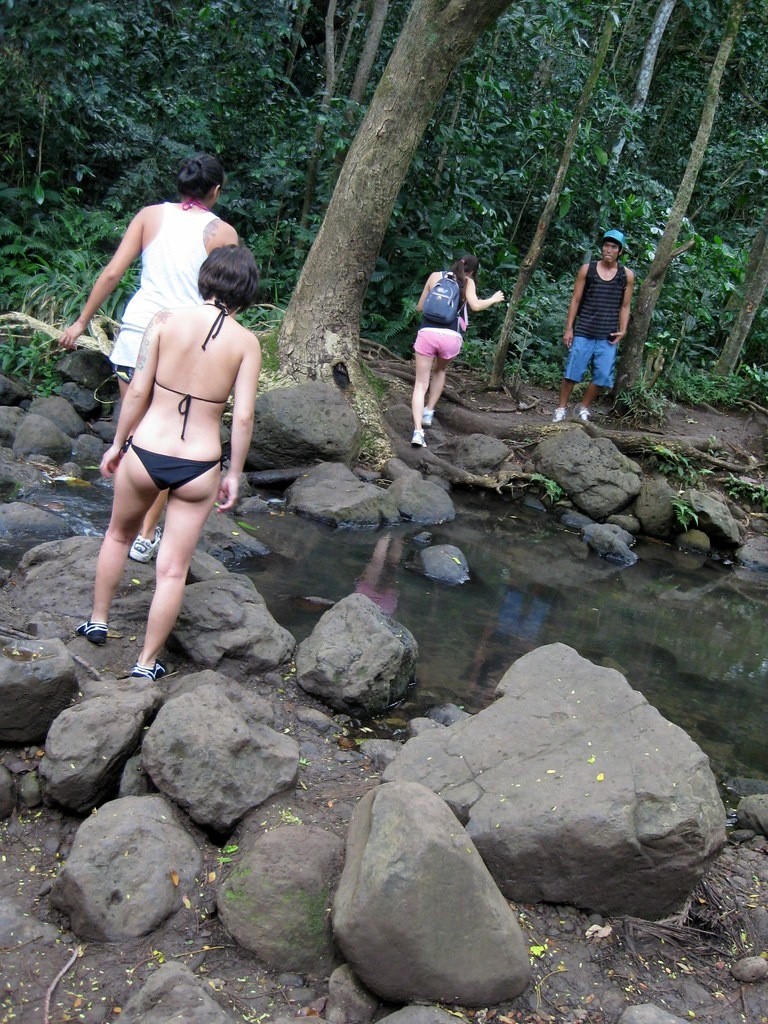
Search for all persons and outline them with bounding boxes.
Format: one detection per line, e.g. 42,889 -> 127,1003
74,245 -> 261,680
552,230 -> 635,422
302,529 -> 407,618
410,253 -> 505,447
59,153 -> 239,563
467,584 -> 554,714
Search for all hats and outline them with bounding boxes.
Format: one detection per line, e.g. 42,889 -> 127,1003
602,229 -> 625,249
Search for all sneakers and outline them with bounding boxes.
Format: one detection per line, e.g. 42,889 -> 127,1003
552,403 -> 570,423
129,525 -> 163,563
572,402 -> 591,432
411,429 -> 427,448
75,620 -> 109,645
421,405 -> 435,426
130,658 -> 187,683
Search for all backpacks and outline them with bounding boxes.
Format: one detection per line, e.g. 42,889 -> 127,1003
423,270 -> 466,324
